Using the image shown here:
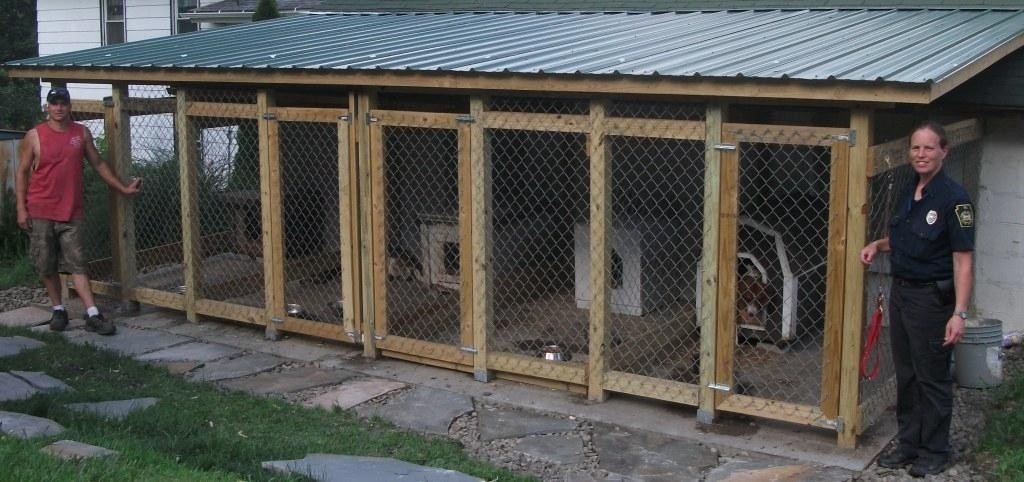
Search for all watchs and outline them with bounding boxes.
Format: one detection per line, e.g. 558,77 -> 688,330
952,311 -> 969,320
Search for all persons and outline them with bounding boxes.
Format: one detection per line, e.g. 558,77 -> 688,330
860,120 -> 975,478
15,87 -> 144,335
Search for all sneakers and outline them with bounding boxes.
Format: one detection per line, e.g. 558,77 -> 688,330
877,445 -> 952,477
49,309 -> 69,331
85,314 -> 116,336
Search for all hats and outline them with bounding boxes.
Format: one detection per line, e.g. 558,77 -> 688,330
47,87 -> 70,102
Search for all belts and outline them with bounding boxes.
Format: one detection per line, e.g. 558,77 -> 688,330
892,275 -> 942,291
